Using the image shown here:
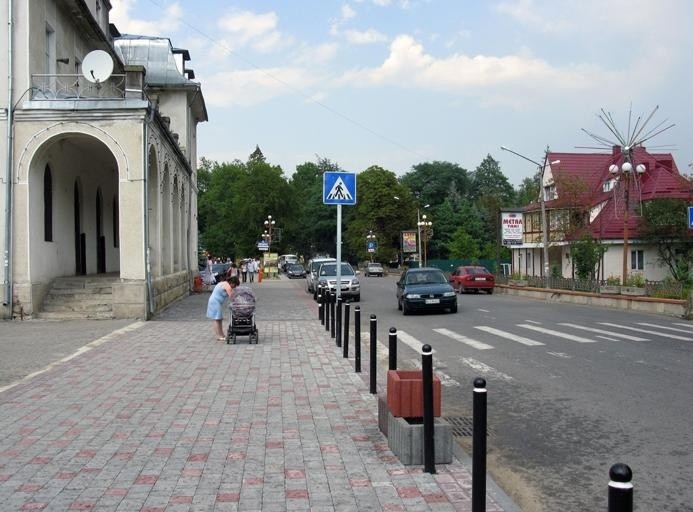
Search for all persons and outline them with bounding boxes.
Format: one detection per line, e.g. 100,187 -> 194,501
203,254 -> 261,290
206,277 -> 241,341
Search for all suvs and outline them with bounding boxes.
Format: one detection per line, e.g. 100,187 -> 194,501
314,262 -> 360,303
199,263 -> 239,285
305,257 -> 337,292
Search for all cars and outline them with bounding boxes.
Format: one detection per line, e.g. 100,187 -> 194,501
449,266 -> 495,296
396,267 -> 459,315
283,263 -> 293,272
288,264 -> 306,280
364,263 -> 383,278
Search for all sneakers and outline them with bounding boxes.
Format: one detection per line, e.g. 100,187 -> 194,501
217,336 -> 226,340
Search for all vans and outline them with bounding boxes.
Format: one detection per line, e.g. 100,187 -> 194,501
280,254 -> 298,266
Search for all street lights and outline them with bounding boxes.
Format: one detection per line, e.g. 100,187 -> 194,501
417,215 -> 434,268
265,216 -> 275,279
608,145 -> 645,286
500,144 -> 560,278
393,196 -> 430,269
367,230 -> 375,263
262,230 -> 270,254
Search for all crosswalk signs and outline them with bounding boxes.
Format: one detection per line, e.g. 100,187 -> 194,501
688,207 -> 693,228
323,172 -> 356,205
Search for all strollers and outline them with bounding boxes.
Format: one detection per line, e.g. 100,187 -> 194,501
225,287 -> 260,346
